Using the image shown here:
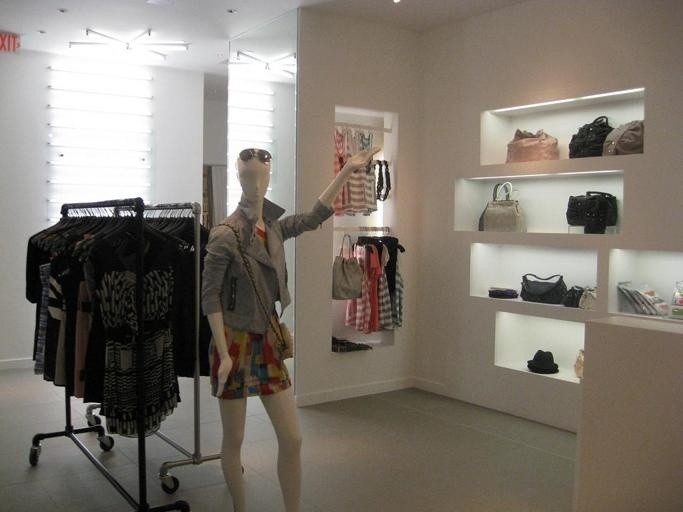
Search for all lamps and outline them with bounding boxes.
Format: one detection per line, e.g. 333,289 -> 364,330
233,49 -> 297,77
67,23 -> 193,62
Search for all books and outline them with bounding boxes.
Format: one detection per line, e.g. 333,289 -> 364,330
617,279 -> 683,320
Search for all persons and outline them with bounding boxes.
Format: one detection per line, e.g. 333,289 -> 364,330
199,145 -> 384,511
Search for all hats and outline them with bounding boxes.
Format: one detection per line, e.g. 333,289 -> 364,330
527,350 -> 558,374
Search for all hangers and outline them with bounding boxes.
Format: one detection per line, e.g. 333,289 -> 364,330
61,200 -> 213,249
335,124 -> 378,138
343,226 -> 406,255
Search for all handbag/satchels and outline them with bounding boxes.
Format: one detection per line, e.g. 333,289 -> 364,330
332,235 -> 361,299
490,274 -> 597,311
506,128 -> 558,163
574,350 -> 584,378
602,119 -> 643,155
273,323 -> 293,360
482,182 -> 526,233
566,191 -> 616,225
568,116 -> 613,158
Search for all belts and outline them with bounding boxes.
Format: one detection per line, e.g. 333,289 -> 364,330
375,160 -> 392,201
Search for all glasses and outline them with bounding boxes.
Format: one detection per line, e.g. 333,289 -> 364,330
239,149 -> 271,161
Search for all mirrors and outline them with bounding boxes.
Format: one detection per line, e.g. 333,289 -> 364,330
227,6 -> 297,393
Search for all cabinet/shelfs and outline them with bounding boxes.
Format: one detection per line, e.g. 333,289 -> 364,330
453,86 -> 682,384
